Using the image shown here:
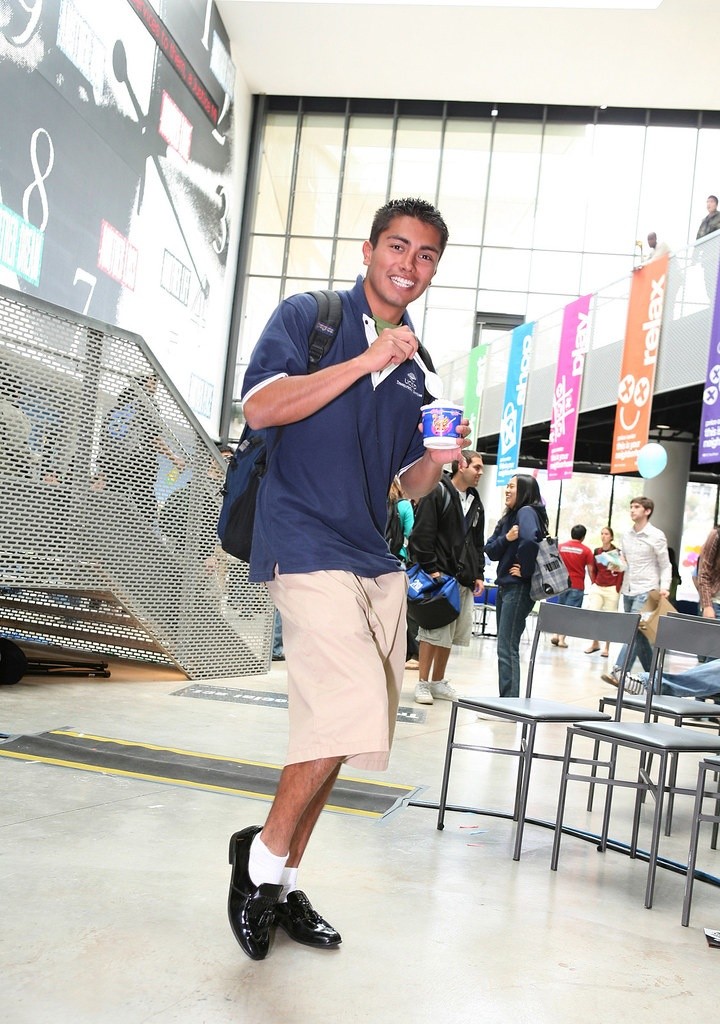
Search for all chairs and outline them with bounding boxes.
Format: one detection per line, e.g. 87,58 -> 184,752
437,602 -> 720,927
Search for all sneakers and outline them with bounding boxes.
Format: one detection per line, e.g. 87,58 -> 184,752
414,679 -> 433,704
430,678 -> 464,701
614,663 -> 647,695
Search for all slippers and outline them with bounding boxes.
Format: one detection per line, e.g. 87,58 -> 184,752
584,648 -> 600,654
601,654 -> 608,657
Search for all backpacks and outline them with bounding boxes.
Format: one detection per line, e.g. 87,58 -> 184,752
216,292 -> 434,563
383,496 -> 408,554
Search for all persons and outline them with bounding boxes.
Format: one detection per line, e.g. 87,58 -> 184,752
229,199 -> 471,961
390,450 -> 485,704
478,474 -> 546,723
602,496 -> 672,686
668,547 -> 681,585
633,232 -> 683,323
101,377 -> 284,662
585,527 -> 623,657
613,658 -> 720,698
692,527 -> 720,725
692,195 -> 720,301
552,524 -> 593,648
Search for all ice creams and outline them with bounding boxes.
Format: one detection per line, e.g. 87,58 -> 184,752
429,398 -> 456,406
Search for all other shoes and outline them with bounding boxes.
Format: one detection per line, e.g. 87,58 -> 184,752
552,639 -> 558,645
558,644 -> 568,648
602,673 -> 618,686
405,659 -> 419,670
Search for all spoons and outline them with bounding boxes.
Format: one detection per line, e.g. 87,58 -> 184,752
413,352 -> 444,398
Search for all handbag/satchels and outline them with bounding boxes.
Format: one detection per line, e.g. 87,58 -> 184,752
639,589 -> 678,643
529,506 -> 572,601
404,562 -> 460,630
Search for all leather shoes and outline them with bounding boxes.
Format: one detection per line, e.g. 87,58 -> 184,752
276,890 -> 343,946
227,823 -> 283,961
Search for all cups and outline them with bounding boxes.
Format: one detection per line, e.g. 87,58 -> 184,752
420,404 -> 465,450
